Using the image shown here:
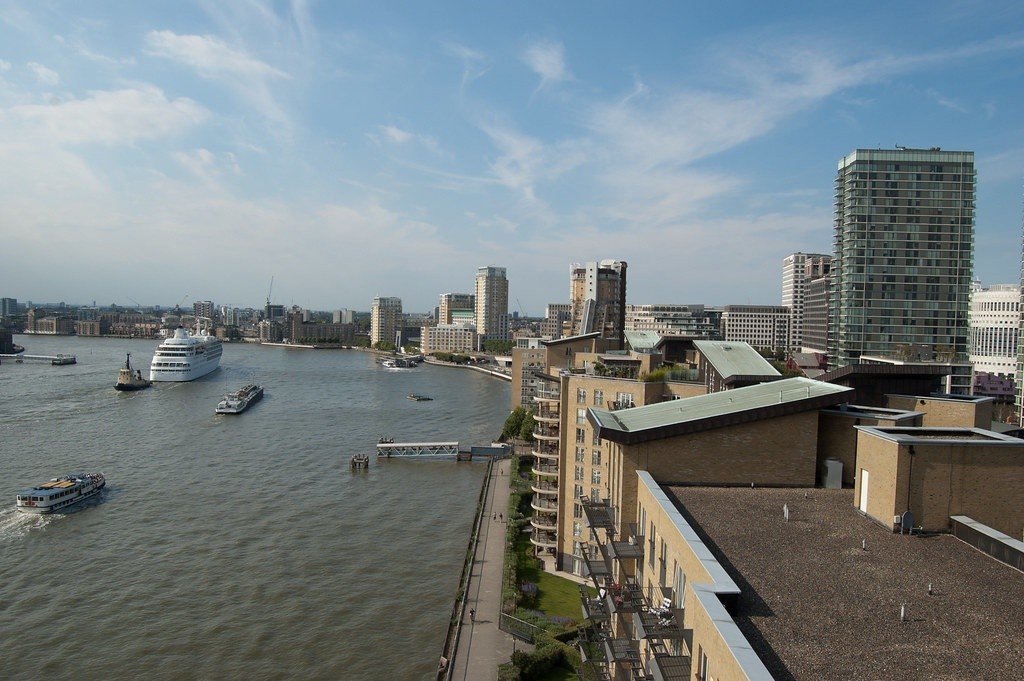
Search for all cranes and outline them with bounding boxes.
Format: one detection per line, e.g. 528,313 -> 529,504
170,296 -> 187,315
266,275 -> 275,320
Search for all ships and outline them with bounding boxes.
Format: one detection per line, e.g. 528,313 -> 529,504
149,320 -> 223,382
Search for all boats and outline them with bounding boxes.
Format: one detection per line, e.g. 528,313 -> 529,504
376,353 -> 418,370
16,359 -> 24,363
215,383 -> 264,415
113,352 -> 151,391
16,472 -> 106,515
52,352 -> 77,365
